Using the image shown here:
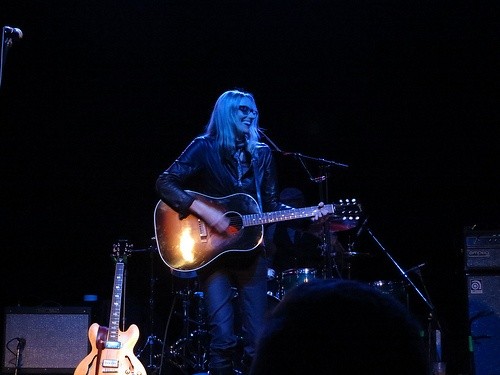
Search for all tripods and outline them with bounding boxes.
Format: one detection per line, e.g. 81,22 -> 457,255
133,246 -> 163,368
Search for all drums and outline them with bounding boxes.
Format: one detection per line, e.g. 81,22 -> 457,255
136,128 -> 439,375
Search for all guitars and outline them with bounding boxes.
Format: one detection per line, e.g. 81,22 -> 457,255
73,241 -> 147,375
154,190 -> 365,273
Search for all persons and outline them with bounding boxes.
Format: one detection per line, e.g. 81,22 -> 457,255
157,90 -> 333,375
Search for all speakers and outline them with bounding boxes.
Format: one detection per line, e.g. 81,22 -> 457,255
466,273 -> 500,375
0,307 -> 91,374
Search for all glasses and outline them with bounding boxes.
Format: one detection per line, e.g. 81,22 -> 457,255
239,105 -> 259,117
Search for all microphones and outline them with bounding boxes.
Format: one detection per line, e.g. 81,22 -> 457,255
357,219 -> 368,237
17,338 -> 24,345
